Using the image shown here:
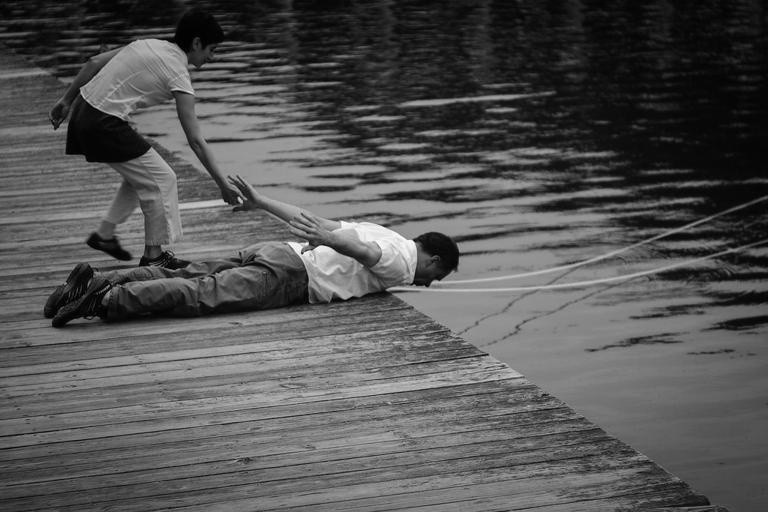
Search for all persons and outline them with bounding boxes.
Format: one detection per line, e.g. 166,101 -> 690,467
47,5 -> 243,270
42,171 -> 464,331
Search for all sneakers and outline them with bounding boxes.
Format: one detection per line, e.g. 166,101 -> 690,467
44,260 -> 114,327
139,250 -> 191,270
86,232 -> 133,261
